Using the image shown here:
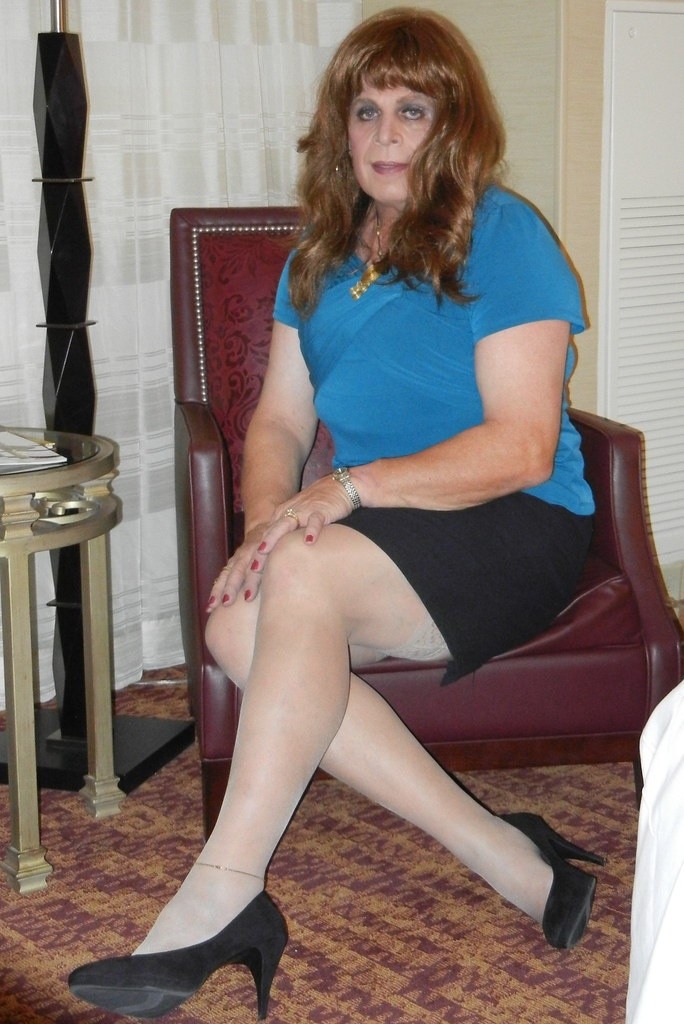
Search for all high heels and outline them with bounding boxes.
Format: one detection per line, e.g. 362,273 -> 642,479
502,812 -> 604,949
67,892 -> 288,1021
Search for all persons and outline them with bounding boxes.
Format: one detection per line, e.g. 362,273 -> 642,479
69,6 -> 605,1021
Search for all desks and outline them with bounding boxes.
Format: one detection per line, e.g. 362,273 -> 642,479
0,429 -> 128,894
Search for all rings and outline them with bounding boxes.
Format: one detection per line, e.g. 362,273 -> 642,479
285,509 -> 301,530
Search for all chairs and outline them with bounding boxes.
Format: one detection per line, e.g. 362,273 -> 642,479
169,204 -> 683,860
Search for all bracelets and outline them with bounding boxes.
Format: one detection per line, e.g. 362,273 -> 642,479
333,465 -> 361,509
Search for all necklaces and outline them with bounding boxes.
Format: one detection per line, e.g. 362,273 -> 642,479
372,205 -> 391,261
